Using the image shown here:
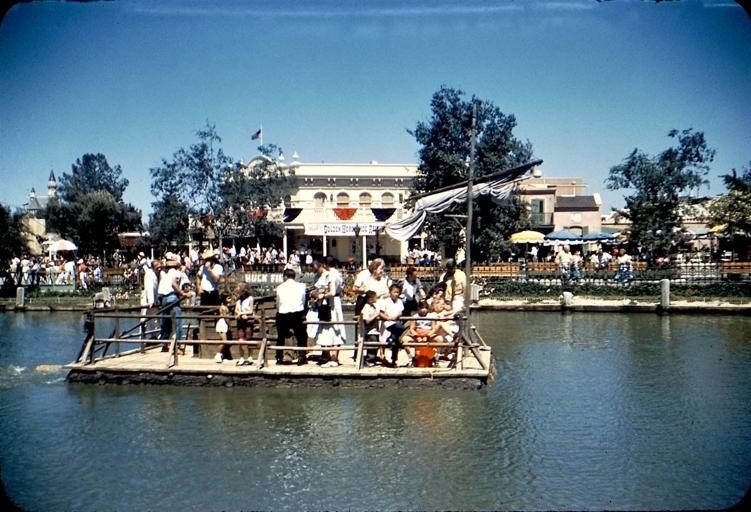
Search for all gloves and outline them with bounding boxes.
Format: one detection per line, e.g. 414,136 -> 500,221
62,311 -> 493,389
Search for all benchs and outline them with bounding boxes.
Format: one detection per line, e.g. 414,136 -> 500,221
251,128 -> 261,140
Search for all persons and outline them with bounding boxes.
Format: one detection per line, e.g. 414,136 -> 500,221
124,243 -> 466,367
1,244 -> 125,295
510,243 -> 718,286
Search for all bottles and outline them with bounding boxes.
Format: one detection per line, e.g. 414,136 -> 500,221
200,249 -> 221,259
166,260 -> 180,267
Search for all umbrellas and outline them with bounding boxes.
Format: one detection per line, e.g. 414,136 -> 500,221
509,223 -> 729,257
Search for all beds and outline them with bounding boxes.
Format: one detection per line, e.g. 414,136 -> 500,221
509,223 -> 729,257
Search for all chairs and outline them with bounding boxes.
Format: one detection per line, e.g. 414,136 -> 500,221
162,345 -> 340,368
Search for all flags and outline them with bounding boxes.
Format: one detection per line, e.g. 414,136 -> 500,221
251,128 -> 261,140
